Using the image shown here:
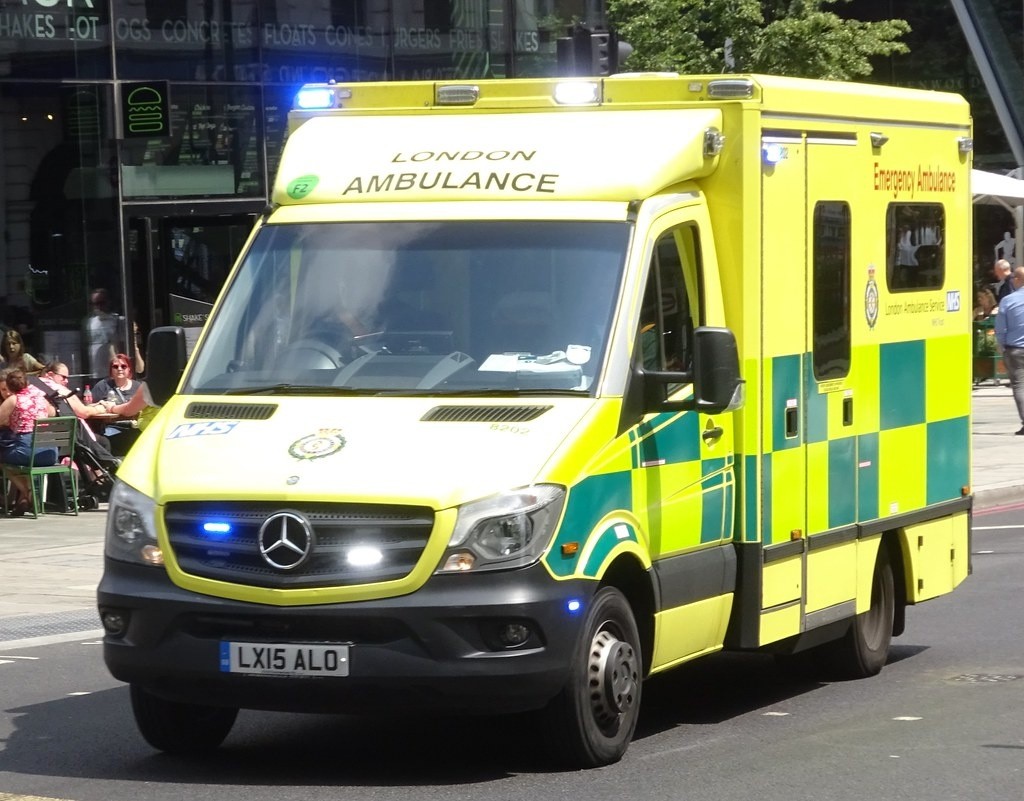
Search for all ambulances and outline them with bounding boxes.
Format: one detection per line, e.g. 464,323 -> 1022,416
92,69 -> 972,770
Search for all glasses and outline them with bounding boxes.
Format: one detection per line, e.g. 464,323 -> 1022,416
55,370 -> 69,380
112,363 -> 129,370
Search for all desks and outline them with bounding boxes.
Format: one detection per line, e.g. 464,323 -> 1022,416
57,411 -> 141,436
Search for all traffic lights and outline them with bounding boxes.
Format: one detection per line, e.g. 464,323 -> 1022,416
590,32 -> 634,76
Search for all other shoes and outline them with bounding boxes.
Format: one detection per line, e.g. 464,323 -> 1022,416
1015,426 -> 1024,435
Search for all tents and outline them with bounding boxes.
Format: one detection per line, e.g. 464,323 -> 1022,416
971,169 -> 1024,217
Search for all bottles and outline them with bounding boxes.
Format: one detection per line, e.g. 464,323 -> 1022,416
83,385 -> 92,406
107,388 -> 115,402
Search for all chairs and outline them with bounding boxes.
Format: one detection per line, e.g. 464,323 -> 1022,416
0,416 -> 79,518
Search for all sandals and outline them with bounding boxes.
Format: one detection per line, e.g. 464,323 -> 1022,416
26,490 -> 40,512
10,497 -> 30,516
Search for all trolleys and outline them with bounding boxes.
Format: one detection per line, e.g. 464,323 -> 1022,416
44,386 -> 123,512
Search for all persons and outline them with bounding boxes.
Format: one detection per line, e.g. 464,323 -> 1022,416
973,259 -> 1024,435
308,278 -> 401,358
0,288 -> 164,516
543,276 -> 660,373
995,232 -> 1016,258
